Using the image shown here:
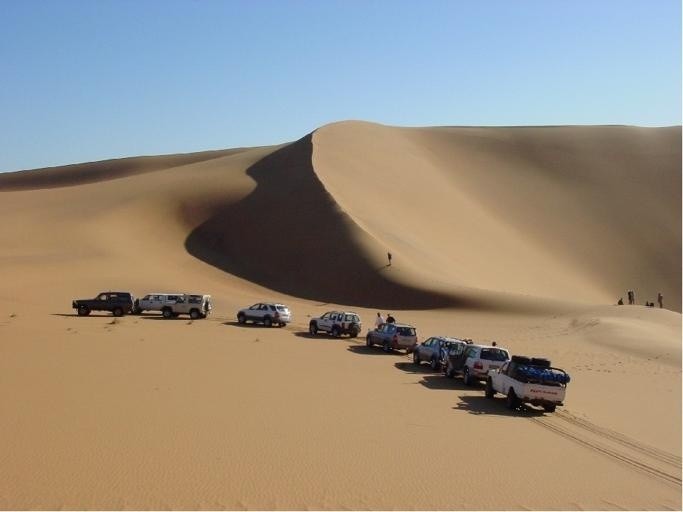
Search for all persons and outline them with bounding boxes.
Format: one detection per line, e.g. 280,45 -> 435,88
617,288 -> 664,308
387,252 -> 392,265
488,341 -> 501,353
385,313 -> 396,325
375,312 -> 384,328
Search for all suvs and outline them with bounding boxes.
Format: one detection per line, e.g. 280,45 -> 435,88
309,310 -> 362,338
73,291 -> 212,319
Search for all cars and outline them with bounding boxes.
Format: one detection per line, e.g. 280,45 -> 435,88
366,322 -> 510,412
238,302 -> 291,328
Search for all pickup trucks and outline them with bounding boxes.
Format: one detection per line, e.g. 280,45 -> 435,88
486,360 -> 571,411
